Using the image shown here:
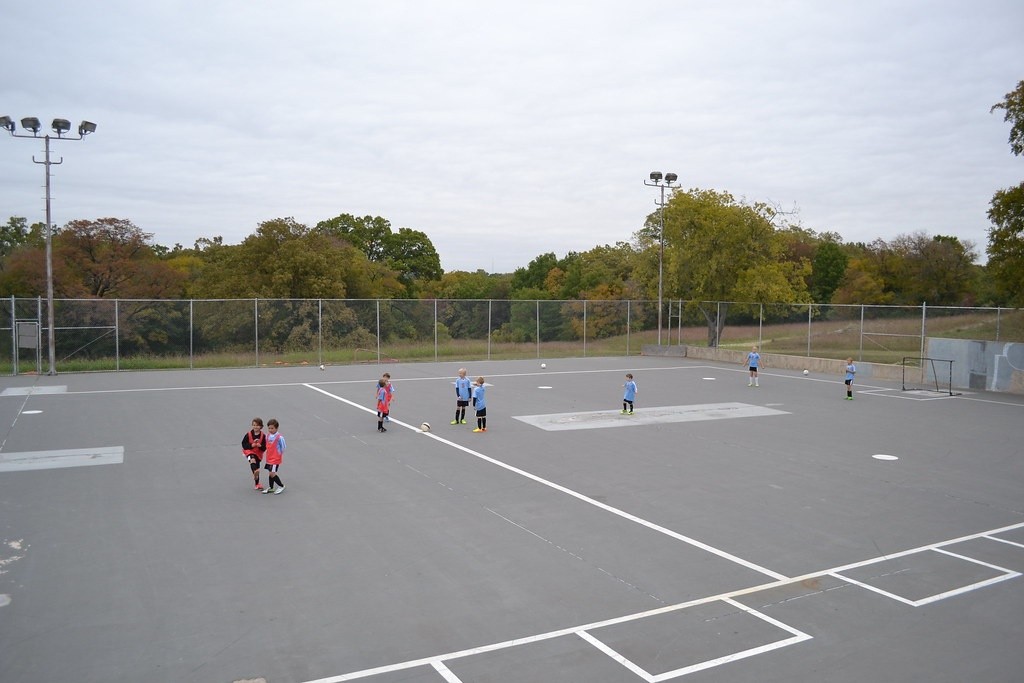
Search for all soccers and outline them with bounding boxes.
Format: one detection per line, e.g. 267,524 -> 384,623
421,422 -> 430,432
803,369 -> 808,375
541,364 -> 546,368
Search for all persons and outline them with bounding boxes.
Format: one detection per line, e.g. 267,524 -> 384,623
844,357 -> 856,400
620,374 -> 638,415
241,418 -> 267,490
471,377 -> 486,433
263,418 -> 286,495
742,346 -> 764,387
375,372 -> 395,433
451,368 -> 471,425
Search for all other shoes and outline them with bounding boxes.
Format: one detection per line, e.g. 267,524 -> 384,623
755,384 -> 759,386
481,426 -> 486,431
377,427 -> 386,432
473,428 -> 483,432
460,419 -> 466,423
620,409 -> 628,414
844,396 -> 848,399
748,383 -> 753,386
848,396 -> 853,399
627,411 -> 634,414
451,420 -> 458,424
253,469 -> 259,480
255,483 -> 263,490
385,418 -> 389,422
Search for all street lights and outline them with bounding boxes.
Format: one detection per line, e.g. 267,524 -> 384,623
650,170 -> 678,345
0,115 -> 100,376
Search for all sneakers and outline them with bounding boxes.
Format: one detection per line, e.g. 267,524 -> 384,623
274,484 -> 286,494
262,486 -> 274,493
